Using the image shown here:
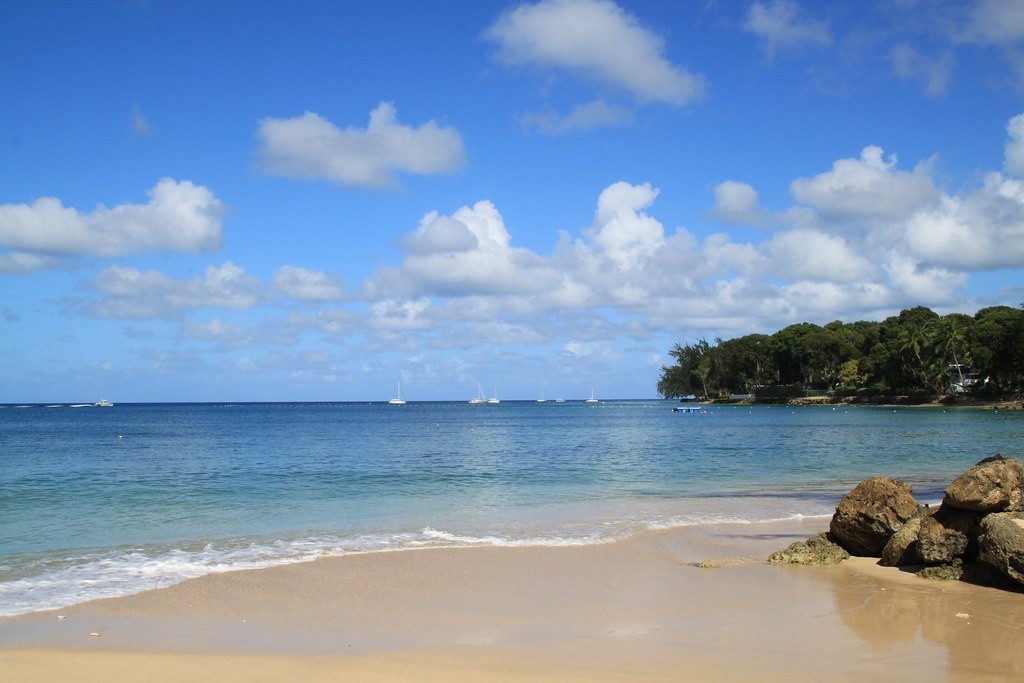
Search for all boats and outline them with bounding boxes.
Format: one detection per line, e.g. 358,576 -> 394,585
95,400 -> 113,408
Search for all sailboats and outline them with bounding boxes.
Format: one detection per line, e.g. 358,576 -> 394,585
389,377 -> 600,404
